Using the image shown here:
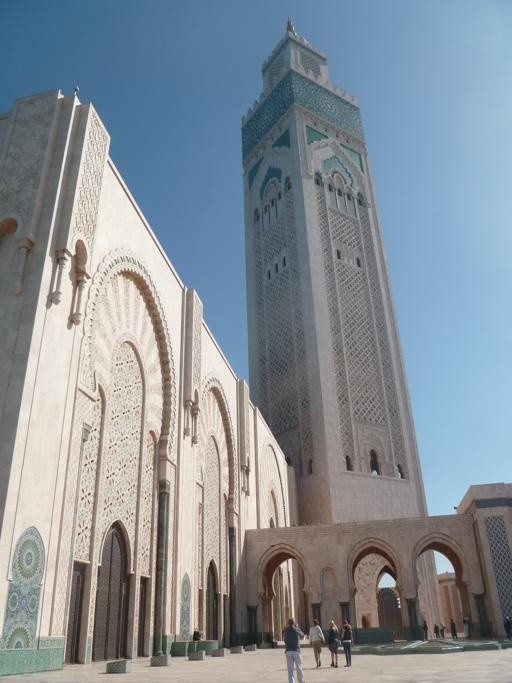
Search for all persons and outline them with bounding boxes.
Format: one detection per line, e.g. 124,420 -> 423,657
309,620 -> 325,667
504,617 -> 512,640
283,619 -> 305,683
326,620 -> 340,667
193,631 -> 204,641
424,619 -> 457,640
341,620 -> 353,667
